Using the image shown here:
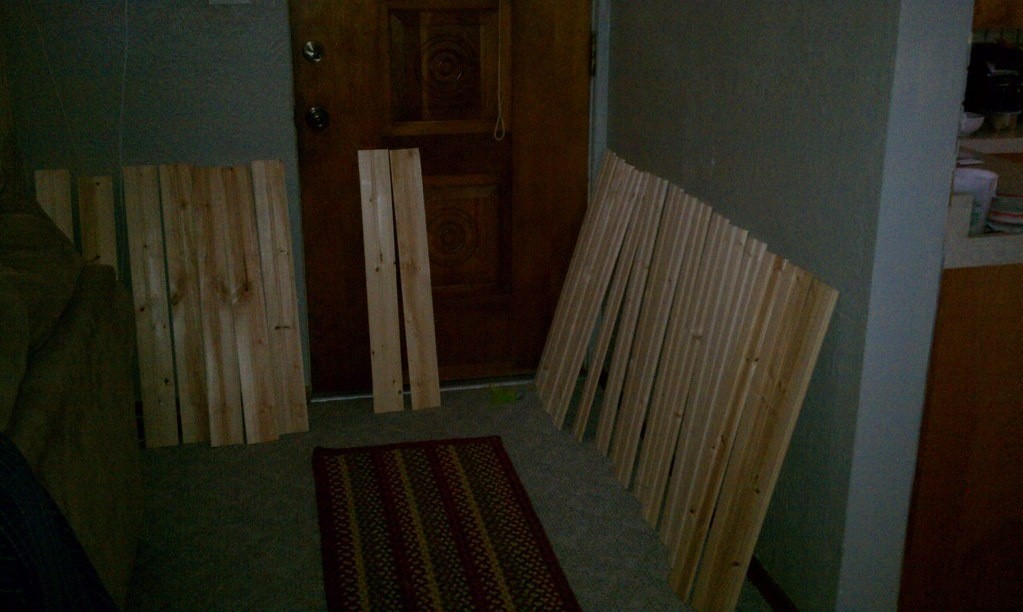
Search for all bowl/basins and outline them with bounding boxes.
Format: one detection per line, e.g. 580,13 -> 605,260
958,111 -> 985,137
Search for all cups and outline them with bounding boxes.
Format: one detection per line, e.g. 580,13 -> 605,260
952,168 -> 999,237
991,107 -> 1021,135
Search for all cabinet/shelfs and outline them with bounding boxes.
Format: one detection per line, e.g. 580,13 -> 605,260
939,0 -> 1023,269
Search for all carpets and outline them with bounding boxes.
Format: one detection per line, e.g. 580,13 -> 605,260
311,435 -> 584,612
